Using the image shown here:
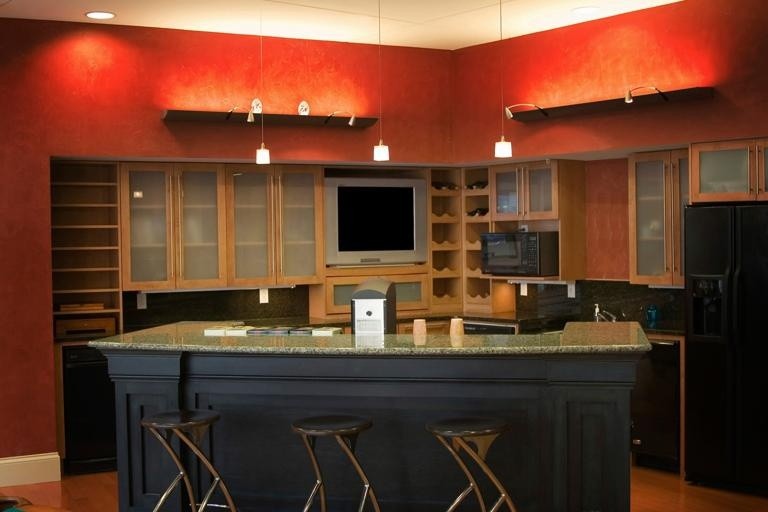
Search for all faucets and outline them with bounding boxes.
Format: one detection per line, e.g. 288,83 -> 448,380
593,303 -> 617,324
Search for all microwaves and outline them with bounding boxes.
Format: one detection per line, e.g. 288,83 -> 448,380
480,230 -> 559,276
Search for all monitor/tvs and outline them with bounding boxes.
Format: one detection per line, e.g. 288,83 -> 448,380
323,178 -> 427,269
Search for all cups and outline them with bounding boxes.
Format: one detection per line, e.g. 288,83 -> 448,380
450,318 -> 464,338
413,319 -> 426,336
450,337 -> 464,348
413,335 -> 426,346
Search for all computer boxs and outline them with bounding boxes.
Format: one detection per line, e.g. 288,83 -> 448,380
349,280 -> 397,335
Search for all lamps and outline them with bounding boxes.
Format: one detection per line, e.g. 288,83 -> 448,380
626,87 -> 669,107
222,104 -> 256,125
501,103 -> 546,119
492,0 -> 513,159
373,1 -> 390,162
252,0 -> 272,167
328,107 -> 360,128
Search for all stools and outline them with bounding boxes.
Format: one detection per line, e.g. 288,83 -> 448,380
291,415 -> 383,511
424,418 -> 518,512
143,408 -> 237,511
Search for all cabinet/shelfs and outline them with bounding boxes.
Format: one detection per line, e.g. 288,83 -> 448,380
225,168 -> 322,286
685,137 -> 768,203
627,146 -> 688,285
489,160 -> 564,219
629,336 -> 682,470
52,159 -> 130,346
427,168 -> 461,308
123,164 -> 227,290
462,168 -> 489,319
